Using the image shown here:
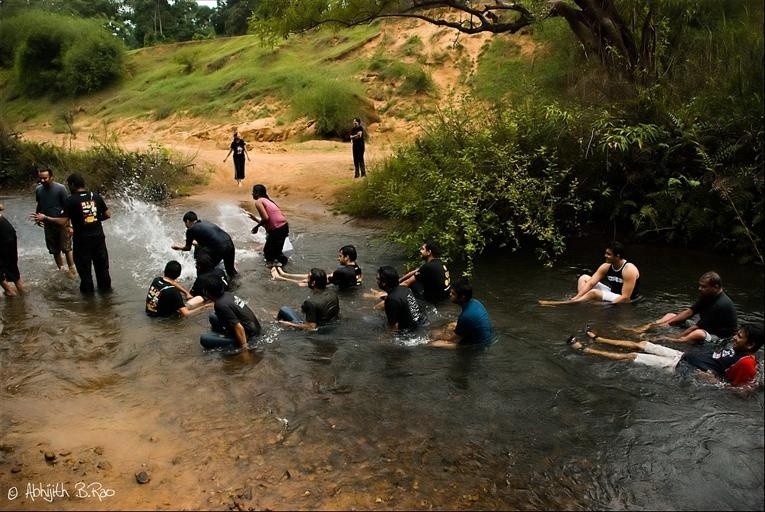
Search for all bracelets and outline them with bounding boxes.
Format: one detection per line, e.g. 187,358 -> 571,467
42,215 -> 48,222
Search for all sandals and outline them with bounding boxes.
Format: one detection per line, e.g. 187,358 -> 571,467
585,326 -> 598,345
567,336 -> 586,355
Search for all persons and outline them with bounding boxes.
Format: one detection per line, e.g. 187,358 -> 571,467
29,173 -> 114,298
35,167 -> 75,278
144,182 -> 500,363
0,205 -> 27,297
539,241 -> 642,308
622,271 -> 738,346
222,132 -> 252,188
348,117 -> 366,179
568,320 -> 765,398
